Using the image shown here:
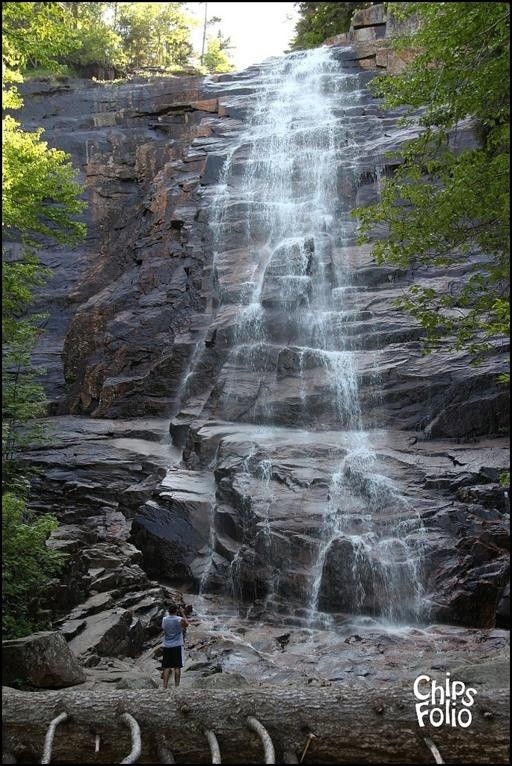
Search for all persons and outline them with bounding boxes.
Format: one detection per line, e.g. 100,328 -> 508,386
161,605 -> 189,689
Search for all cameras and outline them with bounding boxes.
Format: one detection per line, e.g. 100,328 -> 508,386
176,604 -> 187,609
